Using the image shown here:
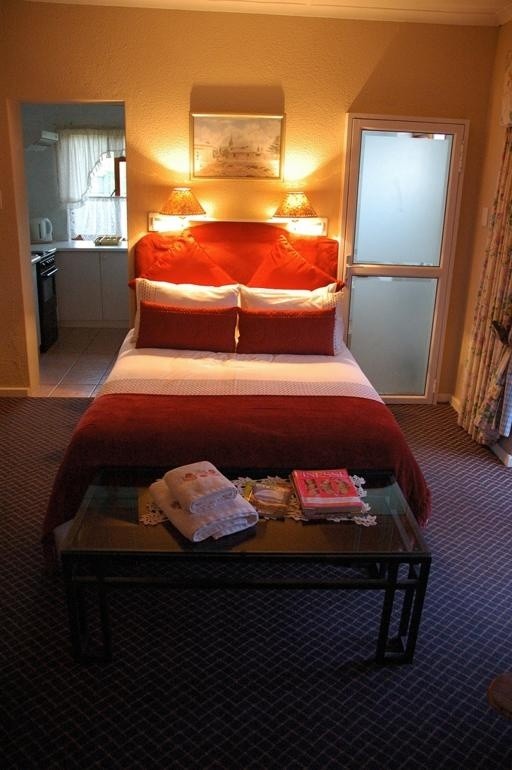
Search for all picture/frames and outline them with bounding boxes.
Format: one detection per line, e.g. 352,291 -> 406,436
189,111 -> 286,184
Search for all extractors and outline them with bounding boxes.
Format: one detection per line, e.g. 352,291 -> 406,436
22,129 -> 59,154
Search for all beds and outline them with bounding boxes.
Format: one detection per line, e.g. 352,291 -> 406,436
41,212 -> 434,574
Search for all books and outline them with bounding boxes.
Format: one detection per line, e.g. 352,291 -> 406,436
246,481 -> 293,518
289,466 -> 364,519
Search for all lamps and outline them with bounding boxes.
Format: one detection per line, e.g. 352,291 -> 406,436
272,192 -> 319,220
158,187 -> 207,218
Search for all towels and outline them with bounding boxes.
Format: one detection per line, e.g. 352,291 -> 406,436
165,460 -> 239,512
149,476 -> 260,543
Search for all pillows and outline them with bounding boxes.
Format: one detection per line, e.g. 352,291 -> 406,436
243,233 -> 347,293
129,273 -> 239,347
128,234 -> 239,286
235,305 -> 337,356
237,279 -> 348,354
133,298 -> 240,360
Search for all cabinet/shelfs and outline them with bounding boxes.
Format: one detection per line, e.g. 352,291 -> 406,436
57,251 -> 102,322
102,251 -> 128,322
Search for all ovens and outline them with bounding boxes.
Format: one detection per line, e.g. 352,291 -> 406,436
37,266 -> 60,353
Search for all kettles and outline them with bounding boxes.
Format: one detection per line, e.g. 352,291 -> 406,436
30,217 -> 52,240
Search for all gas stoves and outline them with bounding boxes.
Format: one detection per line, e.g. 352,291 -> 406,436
31,250 -> 56,273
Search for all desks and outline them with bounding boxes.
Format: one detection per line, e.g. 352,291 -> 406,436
60,473 -> 434,677
